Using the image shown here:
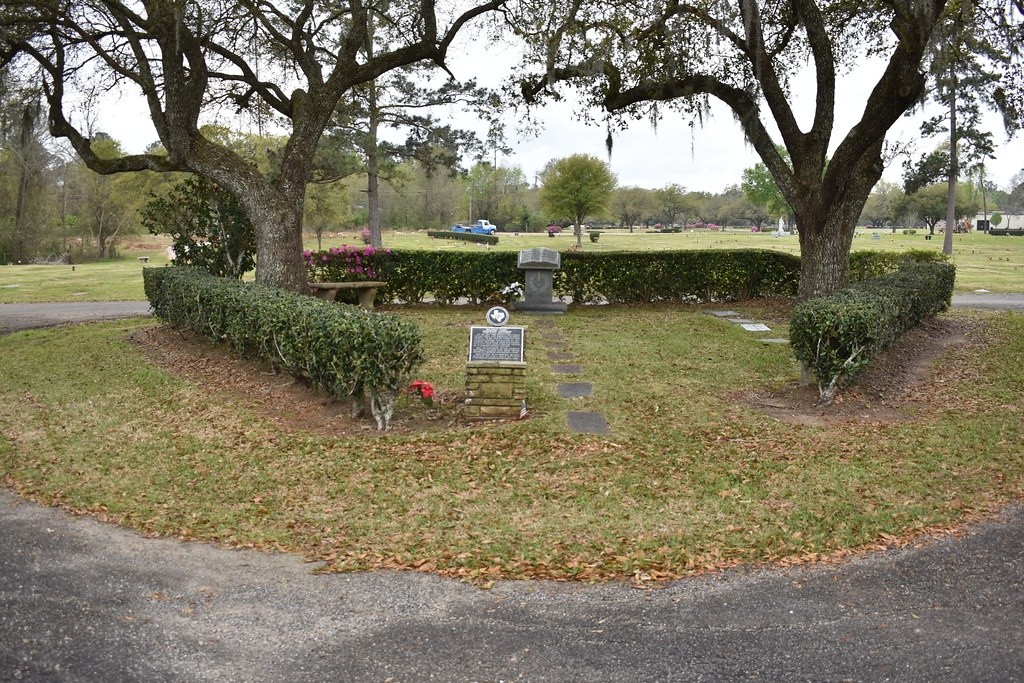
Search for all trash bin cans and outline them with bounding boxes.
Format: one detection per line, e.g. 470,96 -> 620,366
977,219 -> 989,230
549,232 -> 554,237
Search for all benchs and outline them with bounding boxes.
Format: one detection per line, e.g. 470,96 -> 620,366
138,257 -> 150,263
308,281 -> 387,310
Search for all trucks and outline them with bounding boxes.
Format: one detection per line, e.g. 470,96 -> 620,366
453,220 -> 497,235
